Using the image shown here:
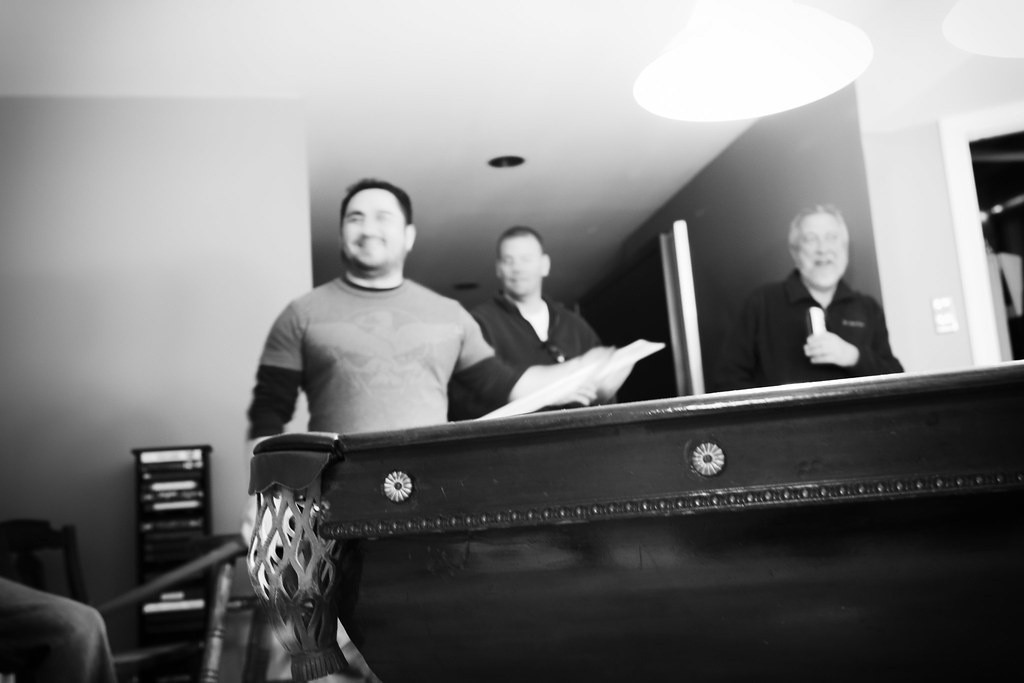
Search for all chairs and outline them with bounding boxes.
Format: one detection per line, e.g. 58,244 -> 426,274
0,518 -> 204,683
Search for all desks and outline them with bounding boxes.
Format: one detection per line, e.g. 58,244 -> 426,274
319,360 -> 1024,683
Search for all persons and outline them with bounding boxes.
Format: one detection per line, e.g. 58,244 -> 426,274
714,204 -> 903,393
247,178 -> 598,683
448,226 -> 618,420
